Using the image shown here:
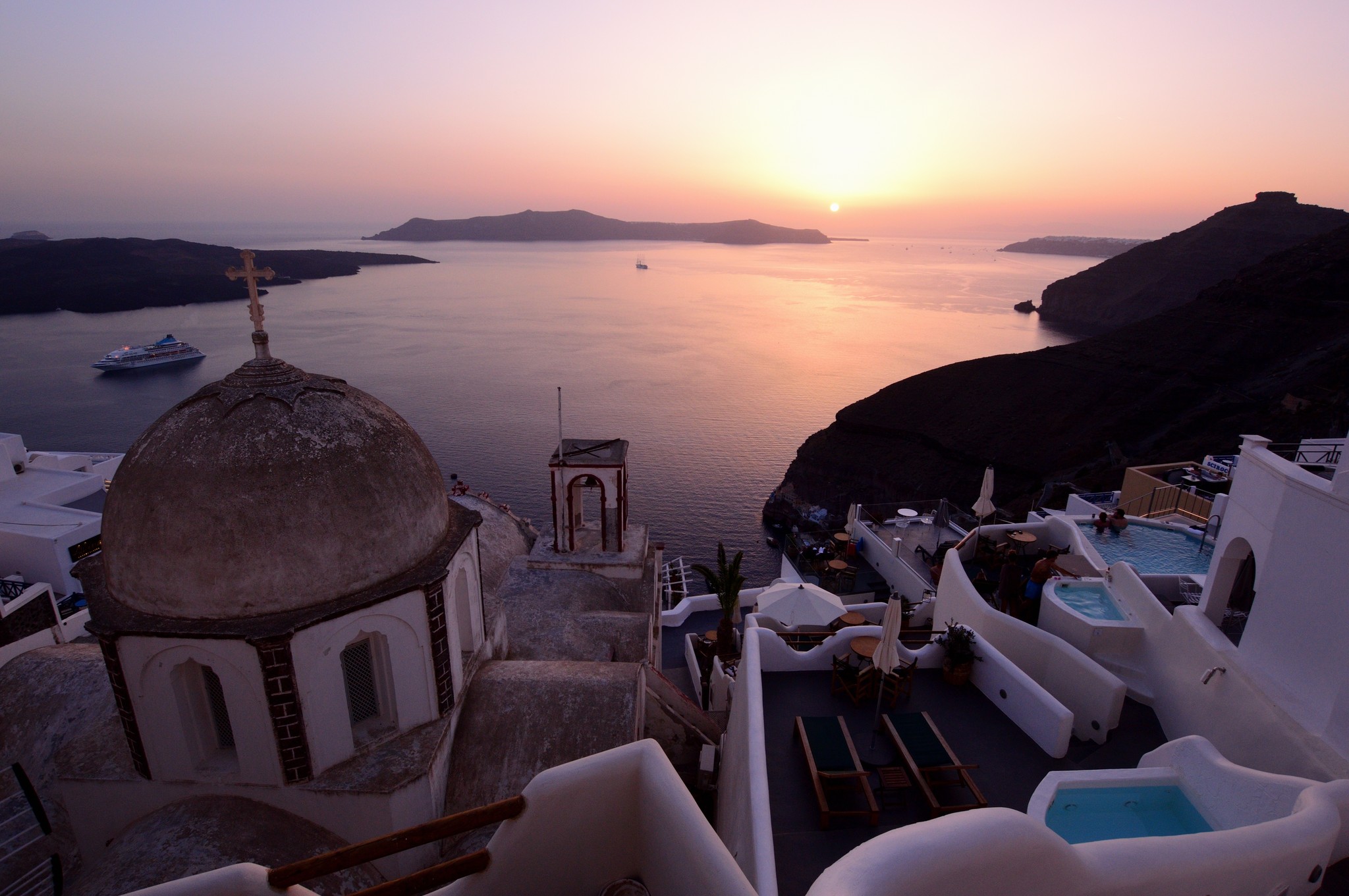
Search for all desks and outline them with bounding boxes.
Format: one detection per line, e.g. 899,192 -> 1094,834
1006,530 -> 1037,562
704,630 -> 718,642
833,532 -> 852,552
876,765 -> 913,813
850,636 -> 881,681
839,611 -> 865,626
897,508 -> 919,531
1188,574 -> 1207,594
1222,460 -> 1233,467
1181,475 -> 1202,488
828,559 -> 848,583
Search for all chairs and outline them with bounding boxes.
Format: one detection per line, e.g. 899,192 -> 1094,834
913,541 -> 956,565
1178,575 -> 1201,606
722,658 -> 738,677
697,638 -> 715,656
893,515 -> 911,538
978,533 -> 1009,569
1217,601 -> 1249,637
793,715 -> 882,832
880,709 -> 989,819
862,618 -> 883,626
1037,543 -> 1071,561
918,509 -> 937,535
872,655 -> 919,709
828,620 -> 852,633
798,531 -> 863,591
830,652 -> 875,708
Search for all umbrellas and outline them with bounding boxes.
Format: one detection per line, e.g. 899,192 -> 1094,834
932,496 -> 950,550
871,590 -> 902,748
970,463 -> 996,559
844,501 -> 856,560
752,602 -> 758,613
756,582 -> 848,651
732,594 -> 742,652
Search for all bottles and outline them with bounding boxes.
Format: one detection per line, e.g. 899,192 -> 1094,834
1105,567 -> 1110,580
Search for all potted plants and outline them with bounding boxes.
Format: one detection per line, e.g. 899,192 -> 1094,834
933,616 -> 984,687
900,610 -> 915,630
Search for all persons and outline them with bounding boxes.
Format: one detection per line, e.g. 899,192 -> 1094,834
1108,509 -> 1128,526
998,550 -> 1022,617
1209,472 -> 1224,479
1190,465 -> 1202,476
1094,512 -> 1111,526
1024,549 -> 1081,613
817,549 -> 835,563
